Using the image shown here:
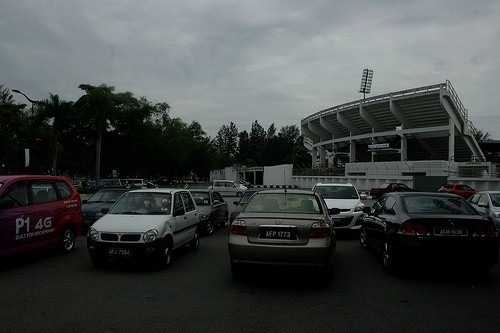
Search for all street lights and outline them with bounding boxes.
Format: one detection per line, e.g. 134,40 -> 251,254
11,89 -> 38,137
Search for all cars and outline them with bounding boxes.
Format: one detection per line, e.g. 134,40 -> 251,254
359,191 -> 497,276
370,183 -> 415,199
440,183 -> 481,200
189,188 -> 229,237
228,189 -> 341,278
303,181 -> 368,241
460,191 -> 500,232
0,175 -> 84,258
229,190 -> 258,230
87,187 -> 201,270
81,188 -> 156,235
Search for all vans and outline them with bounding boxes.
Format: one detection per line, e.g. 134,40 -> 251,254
212,179 -> 248,198
